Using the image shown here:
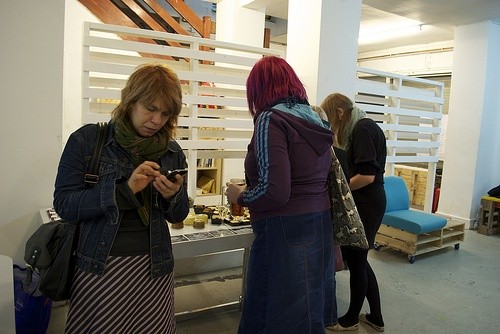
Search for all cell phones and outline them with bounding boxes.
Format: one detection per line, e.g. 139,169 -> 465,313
158,169 -> 188,188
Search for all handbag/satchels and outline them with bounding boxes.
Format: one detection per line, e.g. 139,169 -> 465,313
26,219 -> 77,300
329,146 -> 370,249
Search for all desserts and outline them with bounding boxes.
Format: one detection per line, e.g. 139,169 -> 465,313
172,205 -> 251,229
47,208 -> 59,221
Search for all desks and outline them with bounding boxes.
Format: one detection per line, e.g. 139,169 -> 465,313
39,209 -> 254,323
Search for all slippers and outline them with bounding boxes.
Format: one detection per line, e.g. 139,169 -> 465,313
326,318 -> 360,330
359,313 -> 385,331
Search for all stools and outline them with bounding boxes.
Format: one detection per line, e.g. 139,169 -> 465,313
478,196 -> 500,236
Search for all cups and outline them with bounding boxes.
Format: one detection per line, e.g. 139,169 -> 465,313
171,204 -> 222,229
230,178 -> 245,216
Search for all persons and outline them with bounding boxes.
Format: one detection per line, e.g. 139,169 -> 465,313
53,64 -> 190,334
321,93 -> 387,332
225,56 -> 338,334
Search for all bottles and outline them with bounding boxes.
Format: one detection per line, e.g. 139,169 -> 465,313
473,213 -> 478,231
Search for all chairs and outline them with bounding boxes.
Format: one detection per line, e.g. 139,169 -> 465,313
382,176 -> 448,234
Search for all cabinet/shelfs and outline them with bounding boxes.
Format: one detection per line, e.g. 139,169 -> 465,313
197,157 -> 222,196
374,214 -> 465,263
